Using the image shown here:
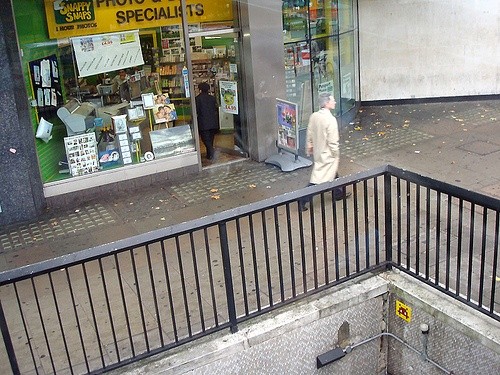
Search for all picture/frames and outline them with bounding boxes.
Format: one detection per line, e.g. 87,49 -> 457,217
111,93 -> 155,166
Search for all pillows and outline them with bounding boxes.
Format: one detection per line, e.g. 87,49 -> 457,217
35,117 -> 53,144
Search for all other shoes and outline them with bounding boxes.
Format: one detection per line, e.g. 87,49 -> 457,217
206,152 -> 214,159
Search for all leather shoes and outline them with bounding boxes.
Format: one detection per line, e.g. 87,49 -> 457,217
302,203 -> 308,211
337,192 -> 352,200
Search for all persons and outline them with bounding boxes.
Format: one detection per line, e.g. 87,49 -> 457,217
195,82 -> 219,160
110,69 -> 131,92
299,93 -> 352,211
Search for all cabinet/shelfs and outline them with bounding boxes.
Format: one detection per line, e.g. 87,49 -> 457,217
159,57 -> 218,120
